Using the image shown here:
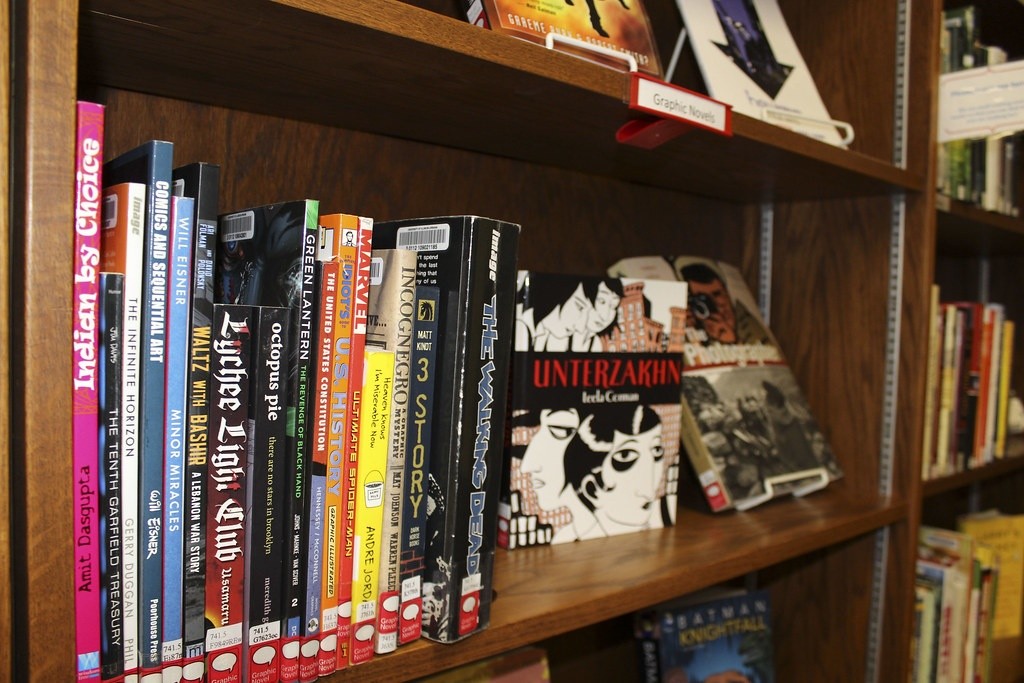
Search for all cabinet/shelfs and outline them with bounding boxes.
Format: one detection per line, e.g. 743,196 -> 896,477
0,0 -> 1024,682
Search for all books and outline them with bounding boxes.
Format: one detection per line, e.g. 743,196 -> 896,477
412,646 -> 550,683
934,5 -> 1024,217
921,284 -> 1015,480
633,587 -> 776,683
608,256 -> 847,516
73,97 -> 521,683
455,0 -> 850,150
907,509 -> 1024,683
497,270 -> 687,550
